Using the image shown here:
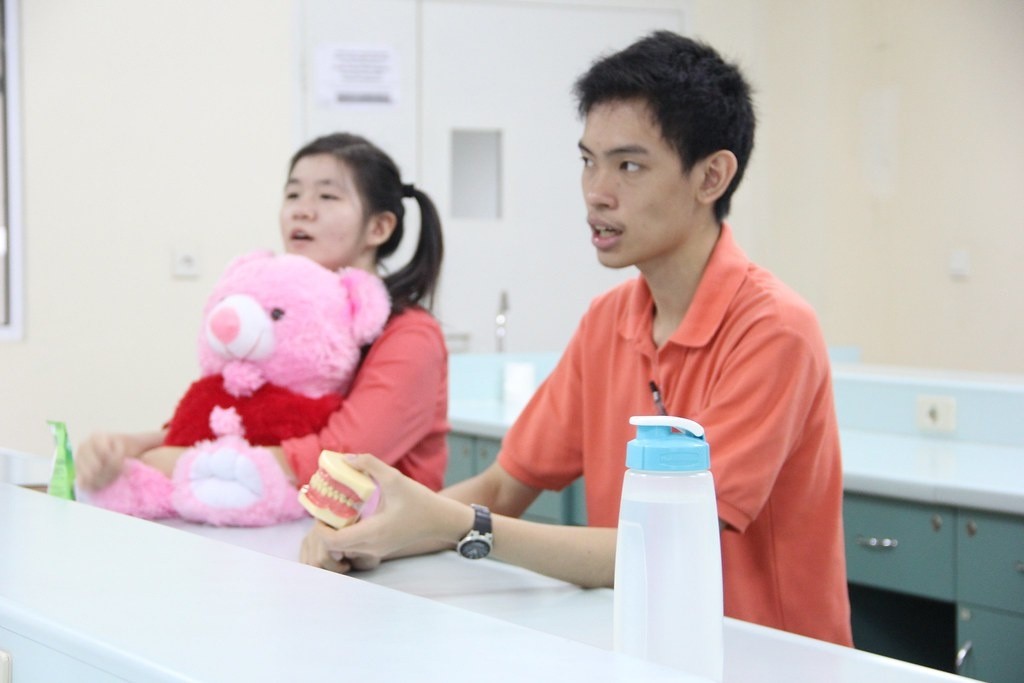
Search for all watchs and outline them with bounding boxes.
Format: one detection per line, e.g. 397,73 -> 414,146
456,504 -> 494,558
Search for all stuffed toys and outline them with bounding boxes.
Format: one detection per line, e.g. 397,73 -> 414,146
91,253 -> 391,529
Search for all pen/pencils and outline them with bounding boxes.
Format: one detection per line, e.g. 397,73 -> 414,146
648,381 -> 667,415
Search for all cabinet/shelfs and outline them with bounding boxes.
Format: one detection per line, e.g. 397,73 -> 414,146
442,433 -> 475,503
478,439 -> 572,523
955,512 -> 1024,683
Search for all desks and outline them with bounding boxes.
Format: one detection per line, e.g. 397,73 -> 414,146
428,587 -> 983,683
150,474 -> 579,592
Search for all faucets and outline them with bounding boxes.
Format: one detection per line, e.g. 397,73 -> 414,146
495,290 -> 510,353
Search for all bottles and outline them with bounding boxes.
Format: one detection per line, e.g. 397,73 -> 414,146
614,415 -> 723,682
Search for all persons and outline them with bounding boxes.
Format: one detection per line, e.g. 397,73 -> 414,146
74,134 -> 448,518
301,30 -> 857,648
